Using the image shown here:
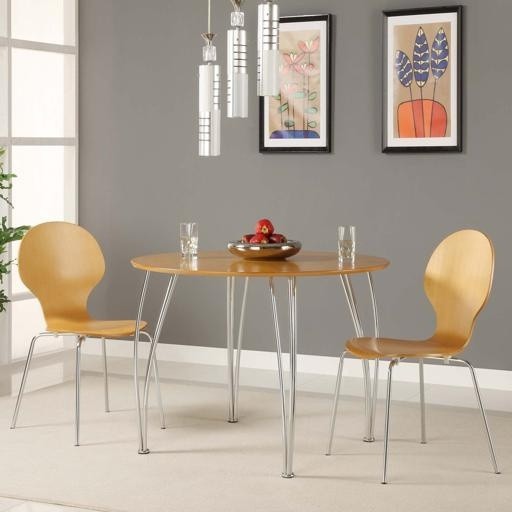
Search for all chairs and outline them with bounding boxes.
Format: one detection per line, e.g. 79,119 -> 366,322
9,221 -> 165,453
325,229 -> 502,479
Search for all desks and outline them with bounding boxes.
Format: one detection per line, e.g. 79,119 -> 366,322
132,250 -> 390,454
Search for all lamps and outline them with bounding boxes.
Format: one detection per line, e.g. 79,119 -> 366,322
198,0 -> 279,157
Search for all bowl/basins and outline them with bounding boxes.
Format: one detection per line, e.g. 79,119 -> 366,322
227,240 -> 301,261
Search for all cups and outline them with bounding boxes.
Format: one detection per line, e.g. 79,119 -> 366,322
180,223 -> 198,258
337,226 -> 355,264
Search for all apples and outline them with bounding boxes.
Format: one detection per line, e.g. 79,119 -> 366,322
242,219 -> 287,244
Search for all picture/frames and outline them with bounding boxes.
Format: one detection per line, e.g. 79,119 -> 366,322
261,4 -> 463,152
